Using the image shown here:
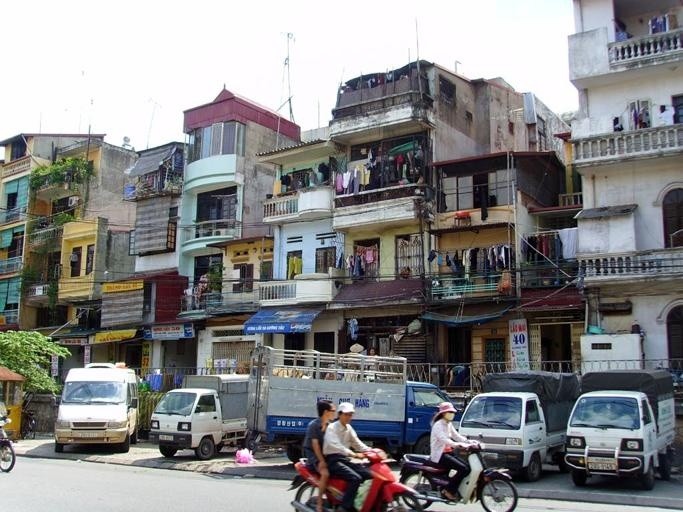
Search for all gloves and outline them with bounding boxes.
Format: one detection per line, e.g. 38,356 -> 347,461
457,443 -> 466,449
468,439 -> 478,443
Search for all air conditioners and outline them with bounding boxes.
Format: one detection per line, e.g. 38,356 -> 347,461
68,196 -> 79,207
212,229 -> 226,236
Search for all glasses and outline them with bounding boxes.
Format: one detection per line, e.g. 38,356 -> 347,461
329,409 -> 336,412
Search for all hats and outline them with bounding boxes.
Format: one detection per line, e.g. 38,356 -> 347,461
337,402 -> 355,413
438,402 -> 457,414
446,367 -> 450,376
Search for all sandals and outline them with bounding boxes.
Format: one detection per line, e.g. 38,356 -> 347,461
316,499 -> 323,511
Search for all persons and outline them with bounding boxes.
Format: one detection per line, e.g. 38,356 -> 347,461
445,366 -> 468,390
430,403 -> 480,501
302,399 -> 337,512
323,402 -> 373,512
370,347 -> 378,357
347,344 -> 364,370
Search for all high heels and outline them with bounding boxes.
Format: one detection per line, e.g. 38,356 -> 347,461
441,489 -> 457,500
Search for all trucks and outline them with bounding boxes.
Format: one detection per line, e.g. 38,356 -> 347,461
458,371 -> 580,482
54,368 -> 140,453
564,369 -> 675,491
400,404 -> 404,405
149,373 -> 250,459
246,346 -> 462,462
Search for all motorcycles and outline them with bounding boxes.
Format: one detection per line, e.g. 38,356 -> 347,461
399,433 -> 518,512
0,402 -> 16,472
287,448 -> 422,512
